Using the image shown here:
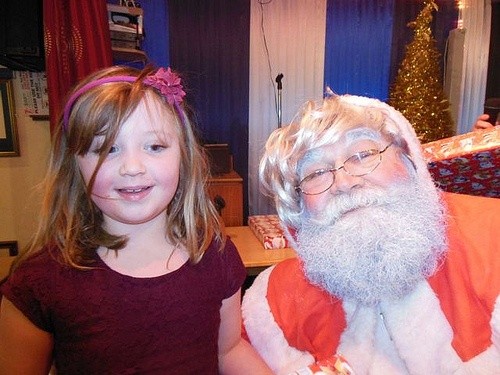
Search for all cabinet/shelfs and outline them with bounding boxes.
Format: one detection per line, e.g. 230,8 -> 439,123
205,170 -> 243,227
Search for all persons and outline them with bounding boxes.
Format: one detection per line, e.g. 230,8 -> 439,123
240,84 -> 500,375
0,65 -> 276,375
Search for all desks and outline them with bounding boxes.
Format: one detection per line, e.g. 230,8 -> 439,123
224,226 -> 297,277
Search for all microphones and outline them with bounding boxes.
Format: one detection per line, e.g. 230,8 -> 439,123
483,96 -> 500,129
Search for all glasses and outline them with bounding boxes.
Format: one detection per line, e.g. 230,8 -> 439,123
294,142 -> 394,195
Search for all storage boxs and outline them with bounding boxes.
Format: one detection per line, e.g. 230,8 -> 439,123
421,123 -> 500,199
248,215 -> 300,250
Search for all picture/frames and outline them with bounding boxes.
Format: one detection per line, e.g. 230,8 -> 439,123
0,78 -> 20,157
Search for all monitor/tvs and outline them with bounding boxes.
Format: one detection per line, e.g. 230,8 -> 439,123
0,0 -> 45,72
